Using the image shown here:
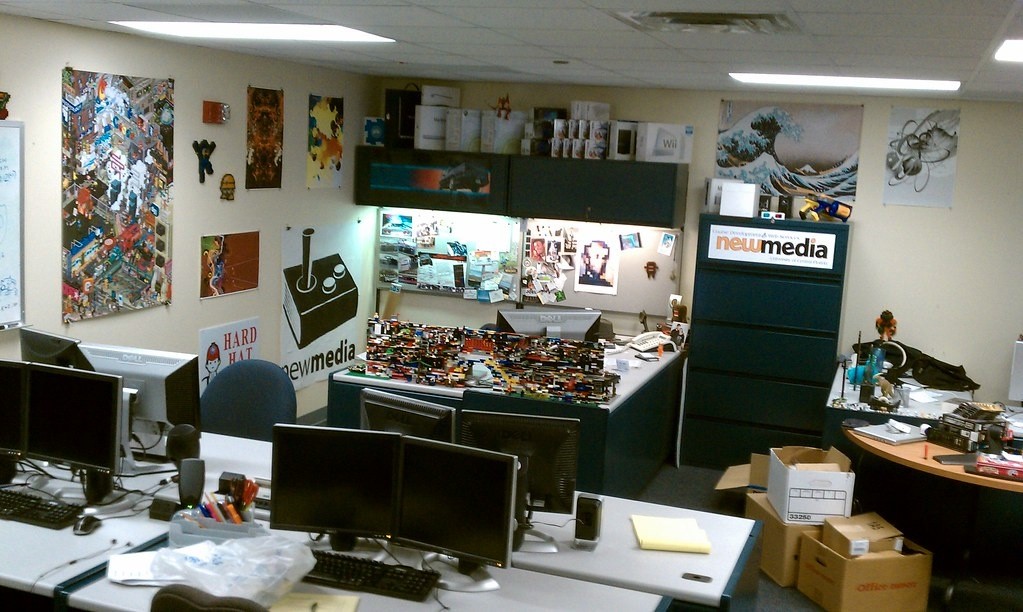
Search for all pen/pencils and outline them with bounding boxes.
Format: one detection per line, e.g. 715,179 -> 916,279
183,514 -> 205,528
198,491 -> 243,523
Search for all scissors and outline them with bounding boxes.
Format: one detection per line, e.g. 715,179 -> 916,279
241,480 -> 260,510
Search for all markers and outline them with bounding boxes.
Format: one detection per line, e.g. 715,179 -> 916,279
0,322 -> 22,331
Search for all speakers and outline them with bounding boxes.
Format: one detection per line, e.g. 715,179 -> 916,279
179,458 -> 205,509
571,493 -> 603,552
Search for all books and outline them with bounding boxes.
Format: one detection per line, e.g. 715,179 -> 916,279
631,514 -> 712,554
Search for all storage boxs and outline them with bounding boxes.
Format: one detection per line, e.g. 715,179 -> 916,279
525,122 -> 554,138
522,139 -> 552,155
446,110 -> 481,150
606,120 -> 639,160
589,121 -> 608,140
745,493 -> 820,587
361,117 -> 385,146
480,110 -> 527,153
413,106 -> 447,150
561,138 -> 571,157
572,139 -> 585,158
796,529 -> 932,612
822,513 -> 903,559
578,120 -> 590,139
767,446 -> 856,526
568,120 -> 579,138
716,453 -> 770,493
528,107 -> 567,122
635,122 -> 696,163
583,139 -> 608,159
571,100 -> 584,119
553,119 -> 568,138
701,177 -> 745,215
720,182 -> 761,218
582,101 -> 610,121
421,85 -> 460,107
825,361 -> 972,421
549,138 -> 561,157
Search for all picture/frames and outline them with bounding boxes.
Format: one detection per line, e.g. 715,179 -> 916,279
0,119 -> 28,330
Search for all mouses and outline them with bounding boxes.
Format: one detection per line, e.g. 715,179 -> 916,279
73,516 -> 101,535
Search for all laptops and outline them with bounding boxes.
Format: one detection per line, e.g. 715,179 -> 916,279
854,422 -> 927,446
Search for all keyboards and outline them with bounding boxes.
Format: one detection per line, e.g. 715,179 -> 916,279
0,489 -> 84,530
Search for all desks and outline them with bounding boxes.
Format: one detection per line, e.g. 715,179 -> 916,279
17,430 -> 273,519
515,491 -> 764,611
462,341 -> 683,501
0,486 -> 182,597
326,362 -> 465,445
845,422 -> 1023,612
60,520 -> 674,612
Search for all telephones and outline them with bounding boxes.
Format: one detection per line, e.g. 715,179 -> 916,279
627,331 -> 677,353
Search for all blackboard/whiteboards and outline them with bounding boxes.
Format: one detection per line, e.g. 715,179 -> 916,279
0,118 -> 26,331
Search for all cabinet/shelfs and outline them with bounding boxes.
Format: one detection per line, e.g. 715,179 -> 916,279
680,213 -> 853,468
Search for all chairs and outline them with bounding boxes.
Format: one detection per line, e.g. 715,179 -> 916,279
200,358 -> 297,441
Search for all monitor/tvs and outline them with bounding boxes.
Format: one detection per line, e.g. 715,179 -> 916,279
392,435 -> 519,592
359,388 -> 456,444
269,423 -> 401,563
0,327 -> 202,516
461,409 -> 581,553
1008,341 -> 1023,401
497,310 -> 602,343
